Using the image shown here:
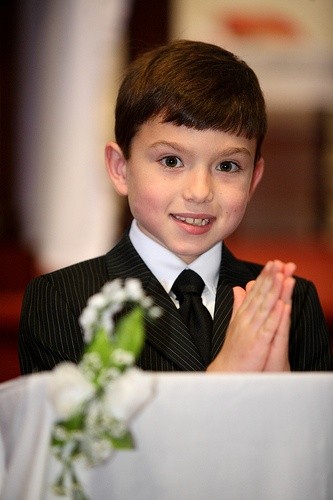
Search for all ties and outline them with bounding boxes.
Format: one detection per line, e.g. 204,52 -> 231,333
172,269 -> 212,367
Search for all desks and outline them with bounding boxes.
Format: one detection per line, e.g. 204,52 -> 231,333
0,371 -> 333,500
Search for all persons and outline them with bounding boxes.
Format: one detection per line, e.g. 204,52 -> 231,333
16,39 -> 333,376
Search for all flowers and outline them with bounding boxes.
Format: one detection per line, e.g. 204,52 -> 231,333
43,278 -> 165,500
